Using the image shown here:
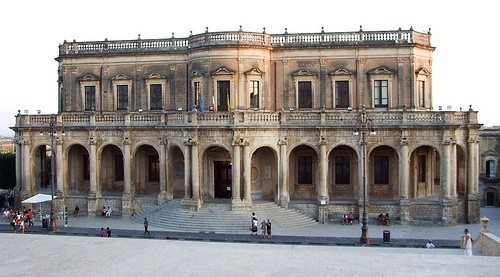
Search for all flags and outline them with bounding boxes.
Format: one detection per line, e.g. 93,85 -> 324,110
227,93 -> 230,112
211,87 -> 215,112
199,95 -> 202,112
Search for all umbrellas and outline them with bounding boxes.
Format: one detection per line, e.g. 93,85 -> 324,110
22,194 -> 57,221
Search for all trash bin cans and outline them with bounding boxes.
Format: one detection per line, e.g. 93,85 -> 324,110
42,218 -> 49,228
383,230 -> 390,244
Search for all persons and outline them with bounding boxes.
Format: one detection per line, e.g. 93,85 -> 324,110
377,213 -> 390,226
73,206 -> 79,217
101,206 -> 111,218
464,229 -> 473,255
342,212 -> 354,225
144,218 -> 150,235
250,213 -> 271,239
3,208 -> 33,234
426,239 -> 435,248
100,227 -> 111,237
